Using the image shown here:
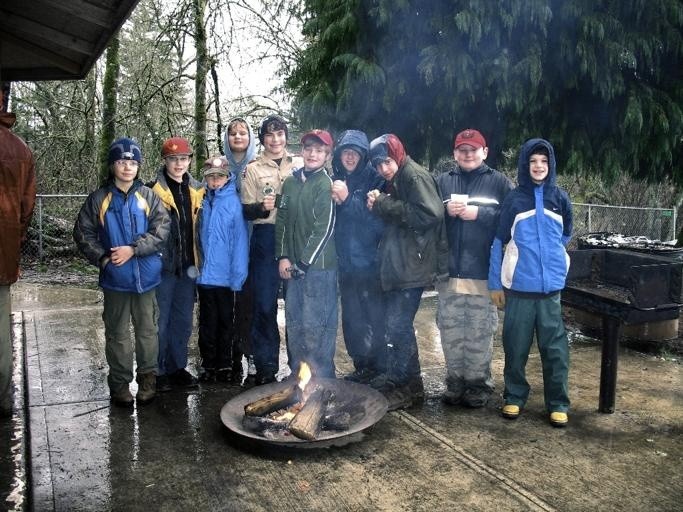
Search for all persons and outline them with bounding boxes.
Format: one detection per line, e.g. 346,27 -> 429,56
330,129 -> 383,379
274,129 -> 339,383
194,156 -> 249,385
486,139 -> 575,427
433,130 -> 512,404
367,133 -> 452,412
144,136 -> 197,382
241,114 -> 304,386
74,138 -> 170,400
1,72 -> 34,423
224,118 -> 259,183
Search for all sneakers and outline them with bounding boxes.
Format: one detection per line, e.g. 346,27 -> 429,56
246,354 -> 258,376
217,359 -> 237,385
465,388 -> 494,409
441,381 -> 464,407
158,375 -> 174,393
384,386 -> 413,411
502,403 -> 520,419
108,383 -> 134,404
173,370 -> 199,388
256,371 -> 275,385
200,358 -> 216,384
453,130 -> 486,153
137,374 -> 158,403
551,410 -> 570,427
408,375 -> 425,398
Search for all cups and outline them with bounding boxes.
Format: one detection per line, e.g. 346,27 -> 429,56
451,193 -> 468,205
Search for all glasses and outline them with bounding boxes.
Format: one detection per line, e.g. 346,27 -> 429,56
164,157 -> 190,163
114,160 -> 140,168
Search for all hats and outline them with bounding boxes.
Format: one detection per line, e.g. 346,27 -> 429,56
107,140 -> 142,163
199,155 -> 230,177
256,113 -> 290,142
301,129 -> 333,150
161,136 -> 193,158
